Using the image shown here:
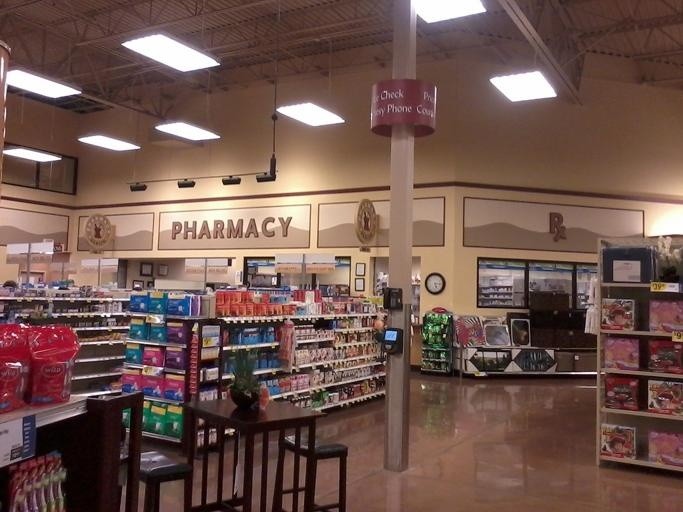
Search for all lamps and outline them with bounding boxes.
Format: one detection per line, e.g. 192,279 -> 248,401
130,183 -> 146,189
222,177 -> 240,185
122,34 -> 220,73
256,174 -> 275,182
412,2 -> 487,22
491,70 -> 555,102
276,102 -> 345,126
3,148 -> 62,162
7,69 -> 81,98
178,180 -> 195,188
77,134 -> 140,152
156,122 -> 220,140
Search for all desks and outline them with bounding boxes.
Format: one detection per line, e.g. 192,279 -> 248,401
181,394 -> 328,512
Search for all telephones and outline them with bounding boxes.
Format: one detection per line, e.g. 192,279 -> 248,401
384,287 -> 402,310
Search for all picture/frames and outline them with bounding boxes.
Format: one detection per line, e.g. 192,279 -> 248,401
455,309 -> 533,345
354,278 -> 366,291
355,263 -> 366,277
132,263 -> 171,290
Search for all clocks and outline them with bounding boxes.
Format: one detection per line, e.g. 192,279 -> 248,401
424,272 -> 445,295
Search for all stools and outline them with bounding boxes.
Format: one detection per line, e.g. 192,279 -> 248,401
284,434 -> 347,511
122,451 -> 193,511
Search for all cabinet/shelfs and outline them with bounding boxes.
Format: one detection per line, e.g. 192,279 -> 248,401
117,289 -> 385,445
592,235 -> 683,473
479,284 -> 514,306
452,343 -> 604,379
2,288 -> 130,392
3,390 -> 145,508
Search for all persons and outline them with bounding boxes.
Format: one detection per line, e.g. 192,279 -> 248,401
205,282 -> 214,295
1,280 -> 18,289
